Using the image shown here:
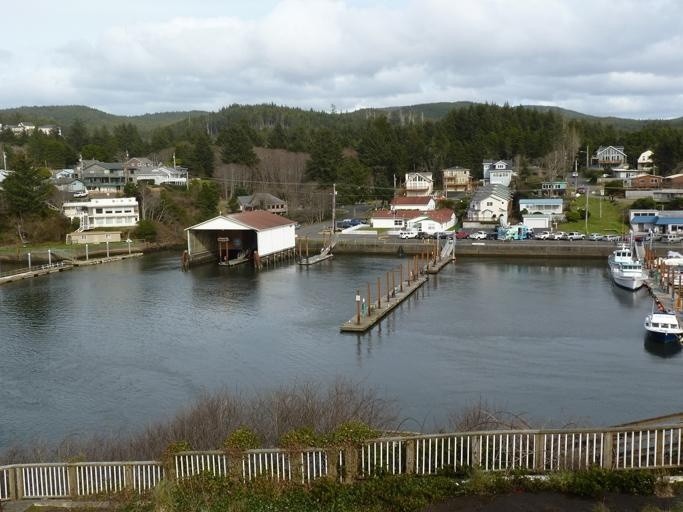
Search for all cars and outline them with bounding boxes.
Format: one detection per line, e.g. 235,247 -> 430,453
572,172 -> 578,177
73,192 -> 88,198
589,233 -> 621,241
419,231 -> 498,241
577,186 -> 587,194
660,234 -> 683,246
535,231 -> 586,240
337,219 -> 362,228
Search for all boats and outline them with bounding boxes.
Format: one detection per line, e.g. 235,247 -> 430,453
608,249 -> 649,290
644,312 -> 683,343
653,252 -> 683,266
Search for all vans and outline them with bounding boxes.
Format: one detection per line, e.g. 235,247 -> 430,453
399,231 -> 418,239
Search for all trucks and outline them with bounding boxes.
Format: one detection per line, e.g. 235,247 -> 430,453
497,225 -> 534,241
